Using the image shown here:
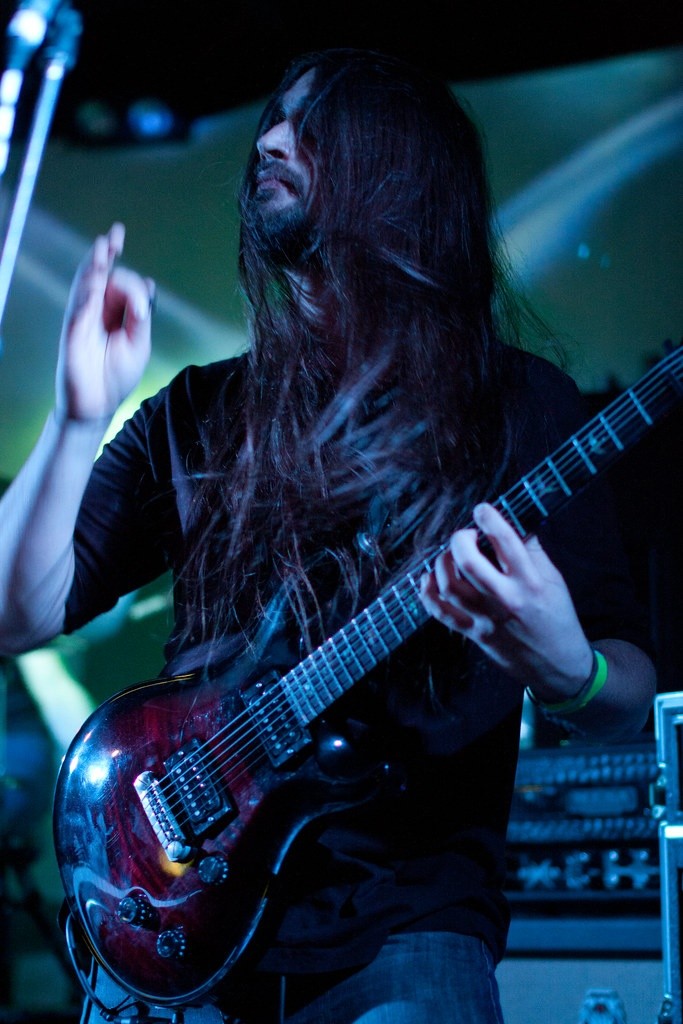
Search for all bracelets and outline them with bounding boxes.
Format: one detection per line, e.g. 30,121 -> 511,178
526,643 -> 610,734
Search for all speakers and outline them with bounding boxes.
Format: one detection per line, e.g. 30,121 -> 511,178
492,917 -> 683,1024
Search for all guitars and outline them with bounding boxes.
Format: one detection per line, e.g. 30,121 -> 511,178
46,342 -> 683,1010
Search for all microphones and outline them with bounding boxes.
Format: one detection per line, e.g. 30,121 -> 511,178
7,0 -> 60,79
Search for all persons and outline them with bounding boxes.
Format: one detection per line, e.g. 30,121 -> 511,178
0,47 -> 660,1024
